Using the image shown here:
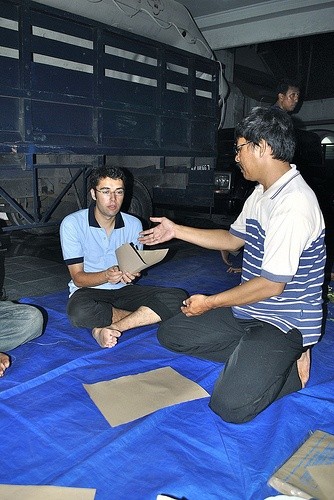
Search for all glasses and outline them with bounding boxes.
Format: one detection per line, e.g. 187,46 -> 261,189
95,188 -> 126,196
234,140 -> 260,153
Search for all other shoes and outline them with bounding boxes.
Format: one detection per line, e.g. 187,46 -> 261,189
221,250 -> 233,266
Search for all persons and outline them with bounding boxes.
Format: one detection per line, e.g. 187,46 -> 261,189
137,79 -> 328,424
59,165 -> 189,348
0,247 -> 43,378
